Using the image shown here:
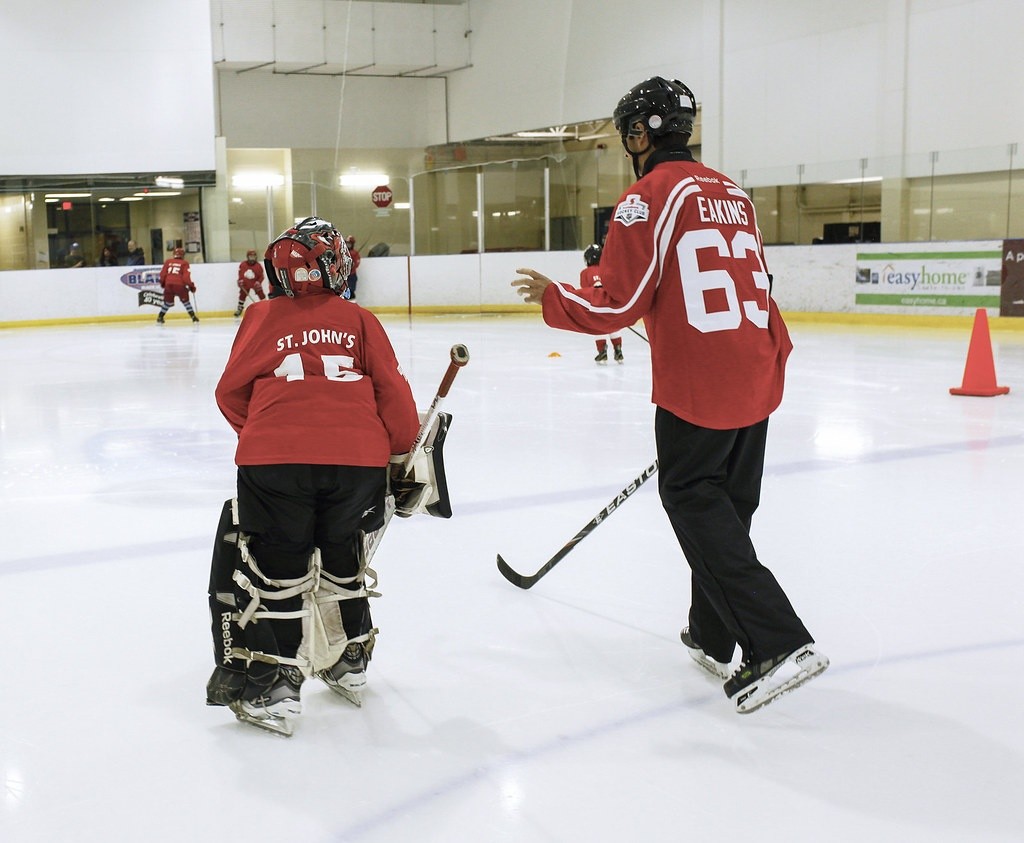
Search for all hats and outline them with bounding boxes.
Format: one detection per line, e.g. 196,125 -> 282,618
72,242 -> 80,249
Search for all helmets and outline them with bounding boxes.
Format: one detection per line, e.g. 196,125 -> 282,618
174,248 -> 185,258
247,251 -> 257,264
264,216 -> 351,296
346,236 -> 355,244
614,75 -> 696,143
584,243 -> 602,265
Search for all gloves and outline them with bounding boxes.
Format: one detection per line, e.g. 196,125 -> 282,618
238,280 -> 245,287
389,452 -> 431,517
190,283 -> 196,292
254,281 -> 262,291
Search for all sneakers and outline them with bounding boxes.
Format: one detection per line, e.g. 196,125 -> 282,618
192,316 -> 200,324
157,316 -> 164,325
723,639 -> 830,714
614,344 -> 625,364
229,665 -> 304,737
316,641 -> 380,707
234,310 -> 242,316
681,626 -> 736,679
595,344 -> 610,366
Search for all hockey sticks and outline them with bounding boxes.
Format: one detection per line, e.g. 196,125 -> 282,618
352,233 -> 372,259
192,281 -> 198,315
363,342 -> 469,568
627,326 -> 650,344
495,458 -> 660,592
242,286 -> 255,303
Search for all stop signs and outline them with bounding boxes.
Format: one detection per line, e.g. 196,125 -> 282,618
372,186 -> 393,207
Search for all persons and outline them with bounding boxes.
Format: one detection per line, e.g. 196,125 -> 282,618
127,240 -> 145,265
64,243 -> 87,268
215,218 -> 433,739
156,248 -> 200,325
94,247 -> 119,267
580,244 -> 624,364
510,76 -> 832,715
342,235 -> 361,304
234,250 -> 267,317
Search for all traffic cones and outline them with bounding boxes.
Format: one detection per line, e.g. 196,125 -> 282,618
949,309 -> 1011,397
